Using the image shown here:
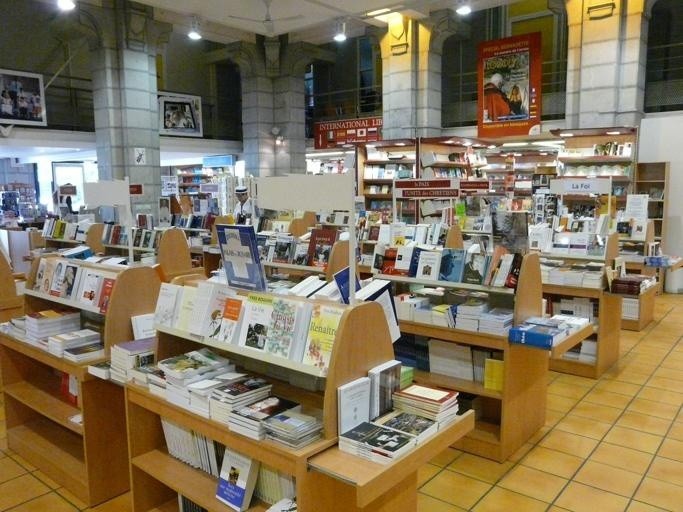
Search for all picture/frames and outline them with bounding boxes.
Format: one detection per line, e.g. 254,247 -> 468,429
0,69 -> 48,127
158,91 -> 204,138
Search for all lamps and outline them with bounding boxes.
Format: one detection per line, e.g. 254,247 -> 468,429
333,20 -> 347,43
56,1 -> 77,13
454,0 -> 471,17
188,21 -> 202,42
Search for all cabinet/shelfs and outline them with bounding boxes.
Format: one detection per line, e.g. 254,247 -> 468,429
572,220 -> 683,279
468,232 -> 661,380
549,128 -> 640,214
488,166 -> 534,195
636,161 -> 672,296
357,225 -> 464,277
260,218 -> 307,237
0,228 -> 32,277
264,212 -> 318,226
0,183 -> 60,231
0,253 -> 164,508
161,172 -> 218,200
168,241 -> 349,304
419,137 -> 490,224
109,274 -> 475,512
153,214 -> 234,277
372,251 -> 596,464
45,222 -> 104,254
0,251 -> 31,323
539,230 -> 661,332
362,138 -> 417,226
96,228 -> 206,282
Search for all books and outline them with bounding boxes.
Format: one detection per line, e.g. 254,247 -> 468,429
181,176 -> 217,183
363,164 -> 412,179
110,284 -> 345,449
187,232 -> 211,247
162,419 -> 225,478
134,252 -> 158,267
421,167 -> 488,182
551,207 -> 608,233
206,224 -> 401,343
257,208 -> 349,232
354,196 -> 452,267
371,244 -> 523,337
59,372 -> 83,427
254,463 -> 296,512
179,187 -> 200,193
532,175 -> 553,186
558,162 -> 633,177
605,257 -> 656,295
541,297 -> 598,323
87,360 -> 111,381
621,298 -> 640,320
645,242 -> 681,268
337,360 -> 459,465
216,446 -> 261,511
528,222 -> 607,256
32,213 -> 97,255
508,314 -> 589,350
174,167 -> 224,174
639,186 -> 664,199
158,194 -> 221,228
454,189 -> 534,256
59,246 -> 129,269
532,194 -> 557,225
191,256 -> 204,267
100,205 -> 128,246
179,492 -> 208,512
0,258 -> 119,364
394,333 -> 503,392
318,158 -> 348,174
367,148 -> 415,160
132,213 -> 164,248
561,339 -> 597,363
558,141 -> 632,157
422,146 -> 487,165
364,183 -> 393,195
615,194 -> 649,262
611,184 -> 633,195
539,257 -> 605,289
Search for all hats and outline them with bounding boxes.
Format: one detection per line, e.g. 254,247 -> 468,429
234,186 -> 247,194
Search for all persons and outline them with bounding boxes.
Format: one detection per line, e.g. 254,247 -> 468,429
234,186 -> 258,225
483,73 -> 521,122
509,85 -> 522,105
